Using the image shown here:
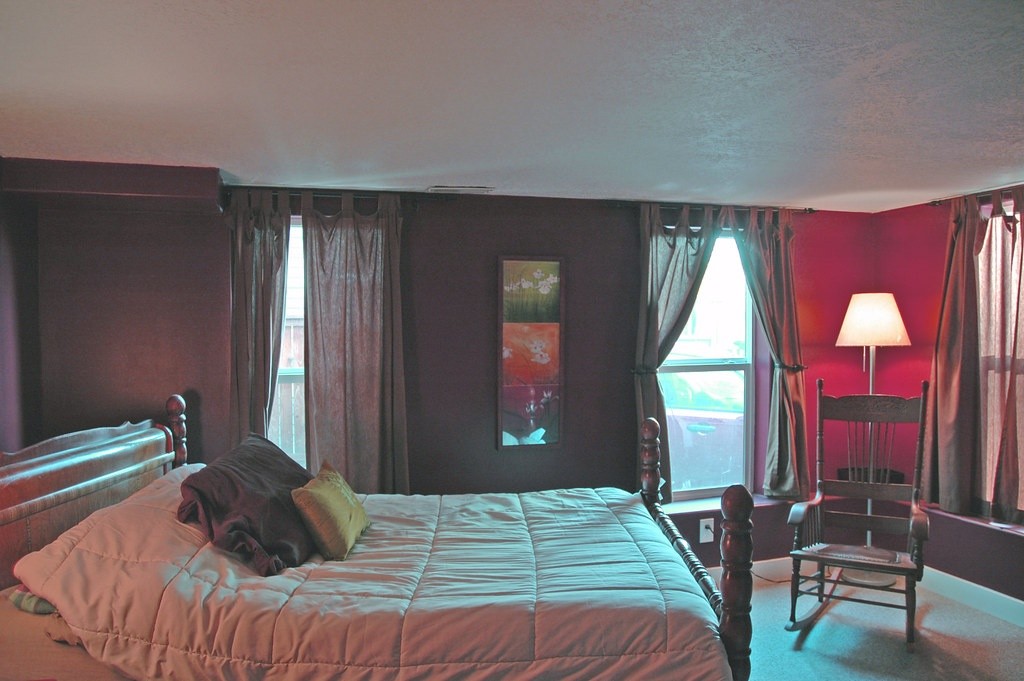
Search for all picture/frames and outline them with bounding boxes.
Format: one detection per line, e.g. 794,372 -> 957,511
495,255 -> 569,449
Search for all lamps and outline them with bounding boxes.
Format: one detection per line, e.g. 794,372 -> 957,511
835,292 -> 912,483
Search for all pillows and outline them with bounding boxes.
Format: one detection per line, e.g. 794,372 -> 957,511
291,457 -> 372,560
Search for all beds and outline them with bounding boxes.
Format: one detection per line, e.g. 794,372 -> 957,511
0,391 -> 753,681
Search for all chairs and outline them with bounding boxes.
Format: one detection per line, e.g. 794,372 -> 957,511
784,379 -> 930,654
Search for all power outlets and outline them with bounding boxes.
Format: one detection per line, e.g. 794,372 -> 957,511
699,518 -> 715,543
